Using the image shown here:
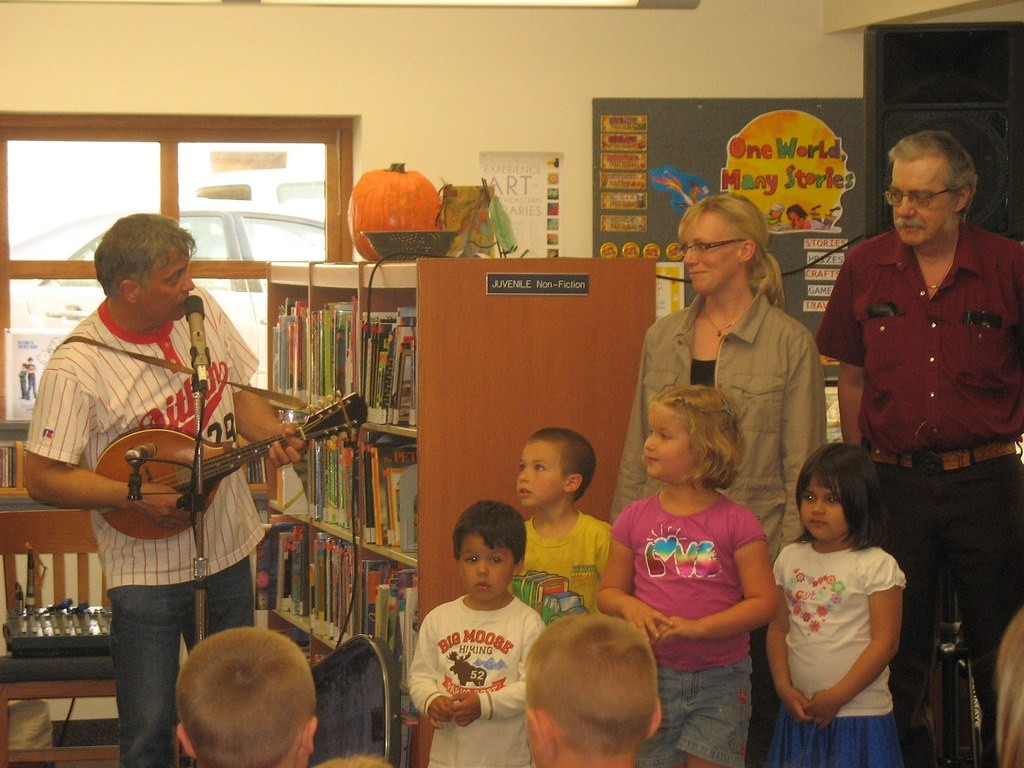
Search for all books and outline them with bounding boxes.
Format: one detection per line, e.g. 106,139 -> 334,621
275,407 -> 418,555
5,331 -> 79,421
1,446 -> 17,489
272,296 -> 416,428
256,514 -> 419,685
265,627 -> 420,768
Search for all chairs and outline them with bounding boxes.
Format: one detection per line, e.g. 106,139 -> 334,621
1,509 -> 127,768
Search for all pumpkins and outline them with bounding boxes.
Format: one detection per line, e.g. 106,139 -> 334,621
346,163 -> 442,261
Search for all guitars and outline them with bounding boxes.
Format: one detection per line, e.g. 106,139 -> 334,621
91,388 -> 369,541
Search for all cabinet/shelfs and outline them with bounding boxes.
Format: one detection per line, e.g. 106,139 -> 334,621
267,259 -> 656,767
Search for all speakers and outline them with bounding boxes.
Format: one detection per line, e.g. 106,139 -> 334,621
862,21 -> 1024,246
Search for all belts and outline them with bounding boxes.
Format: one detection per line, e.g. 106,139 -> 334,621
869,440 -> 1016,470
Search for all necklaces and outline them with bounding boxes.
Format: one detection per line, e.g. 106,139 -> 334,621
702,307 -> 735,337
925,260 -> 953,289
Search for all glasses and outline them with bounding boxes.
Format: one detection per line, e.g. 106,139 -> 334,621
675,239 -> 757,256
882,186 -> 971,208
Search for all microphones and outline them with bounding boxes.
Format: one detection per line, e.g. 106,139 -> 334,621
183,295 -> 209,393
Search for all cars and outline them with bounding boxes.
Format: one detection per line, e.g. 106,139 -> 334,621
29,202 -> 325,387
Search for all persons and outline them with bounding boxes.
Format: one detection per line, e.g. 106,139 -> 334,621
174,614 -> 661,768
18,358 -> 38,399
23,213 -> 308,768
407,385 -> 908,768
815,128 -> 1023,767
608,192 -> 828,767
